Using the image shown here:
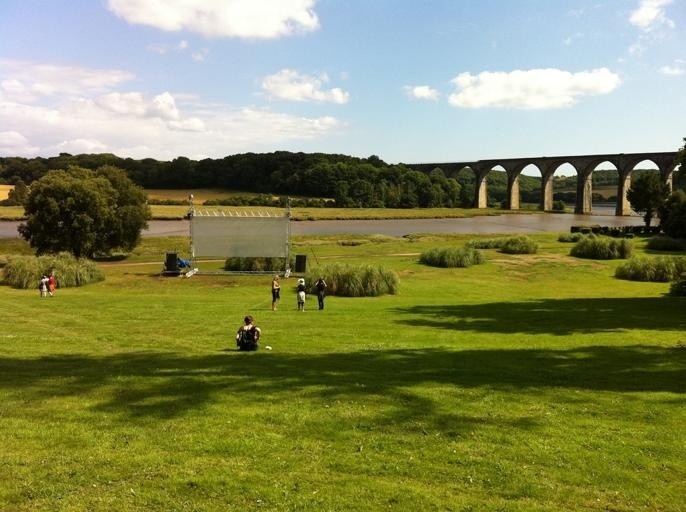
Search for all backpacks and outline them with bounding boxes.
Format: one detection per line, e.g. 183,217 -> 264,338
317,279 -> 325,290
240,325 -> 258,351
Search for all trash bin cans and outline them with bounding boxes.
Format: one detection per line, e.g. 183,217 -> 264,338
166,253 -> 177,271
296,255 -> 306,272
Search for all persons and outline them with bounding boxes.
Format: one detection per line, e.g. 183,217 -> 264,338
38,275 -> 50,298
46,272 -> 56,298
271,274 -> 282,311
296,278 -> 307,313
235,315 -> 262,351
314,277 -> 328,310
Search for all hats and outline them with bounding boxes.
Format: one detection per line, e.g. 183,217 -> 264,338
297,278 -> 305,285
